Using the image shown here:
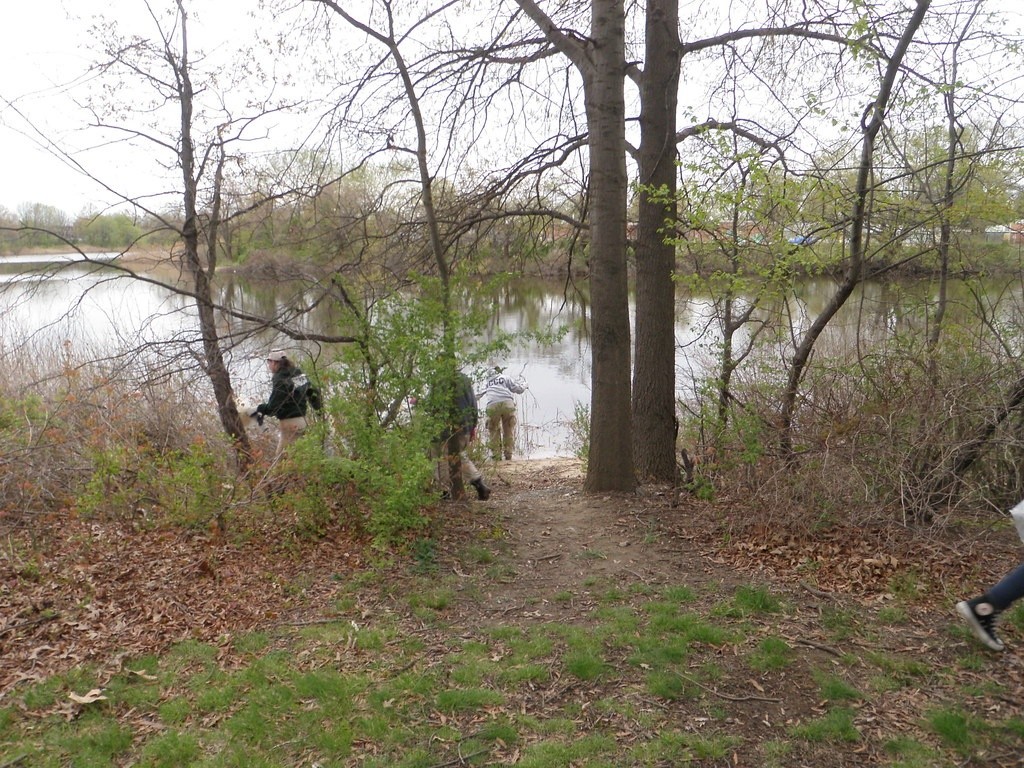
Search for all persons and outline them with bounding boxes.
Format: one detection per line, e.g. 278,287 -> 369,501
473,365 -> 529,461
249,351 -> 323,457
411,350 -> 493,501
956,498 -> 1024,651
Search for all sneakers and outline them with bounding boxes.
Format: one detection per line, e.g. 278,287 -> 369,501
955,592 -> 1006,651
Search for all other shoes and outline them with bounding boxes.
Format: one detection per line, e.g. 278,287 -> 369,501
504,457 -> 512,461
490,454 -> 503,462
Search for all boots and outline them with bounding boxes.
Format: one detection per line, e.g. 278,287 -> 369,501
470,476 -> 491,502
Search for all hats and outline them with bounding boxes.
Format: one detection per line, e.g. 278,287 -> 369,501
263,348 -> 288,361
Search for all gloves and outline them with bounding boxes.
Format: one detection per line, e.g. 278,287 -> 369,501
249,410 -> 265,426
409,397 -> 417,404
469,427 -> 478,442
